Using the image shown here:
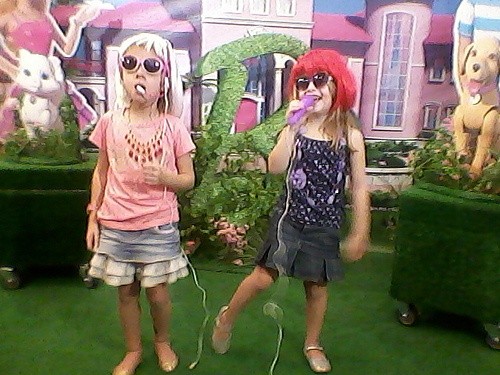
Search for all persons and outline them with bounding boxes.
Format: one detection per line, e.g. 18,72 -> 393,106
87,34 -> 197,375
212,48 -> 372,373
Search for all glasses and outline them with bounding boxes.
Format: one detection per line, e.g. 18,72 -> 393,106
296,70 -> 334,92
119,53 -> 164,74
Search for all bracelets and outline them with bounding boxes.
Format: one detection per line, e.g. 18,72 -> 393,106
87,203 -> 98,213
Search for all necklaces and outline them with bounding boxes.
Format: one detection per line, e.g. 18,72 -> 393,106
124,108 -> 164,164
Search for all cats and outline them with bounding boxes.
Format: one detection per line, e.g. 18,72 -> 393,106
3,48 -> 65,148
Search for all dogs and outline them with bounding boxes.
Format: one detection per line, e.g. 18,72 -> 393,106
454,38 -> 500,181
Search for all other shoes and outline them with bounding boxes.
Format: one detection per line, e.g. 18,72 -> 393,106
152,335 -> 178,371
303,344 -> 330,372
112,347 -> 144,375
211,305 -> 231,353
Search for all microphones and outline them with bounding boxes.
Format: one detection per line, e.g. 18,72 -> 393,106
136,85 -> 149,101
287,95 -> 314,124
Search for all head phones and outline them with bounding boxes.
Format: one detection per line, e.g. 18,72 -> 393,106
164,77 -> 170,98
